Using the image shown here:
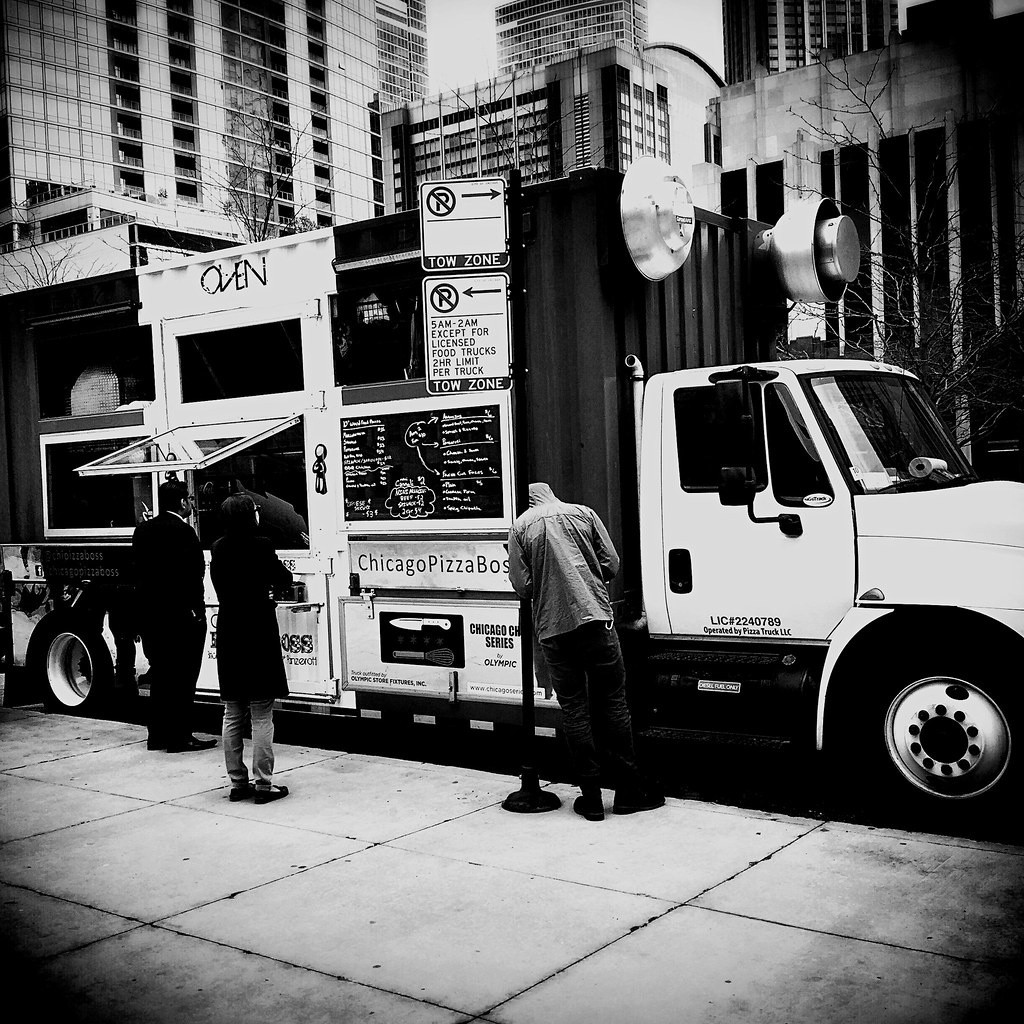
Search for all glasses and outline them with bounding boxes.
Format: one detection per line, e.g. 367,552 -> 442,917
187,494 -> 195,501
251,504 -> 262,514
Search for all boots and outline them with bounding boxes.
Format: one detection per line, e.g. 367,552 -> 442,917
574,774 -> 604,821
613,772 -> 665,815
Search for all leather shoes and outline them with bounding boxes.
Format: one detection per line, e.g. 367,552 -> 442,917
166,735 -> 218,753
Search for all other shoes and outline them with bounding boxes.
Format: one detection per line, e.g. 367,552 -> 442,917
252,785 -> 289,804
229,783 -> 255,802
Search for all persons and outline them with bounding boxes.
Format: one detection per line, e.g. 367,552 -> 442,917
508,482 -> 665,821
209,495 -> 293,805
132,481 -> 218,754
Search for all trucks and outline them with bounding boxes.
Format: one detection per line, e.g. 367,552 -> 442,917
0,158 -> 1024,825
